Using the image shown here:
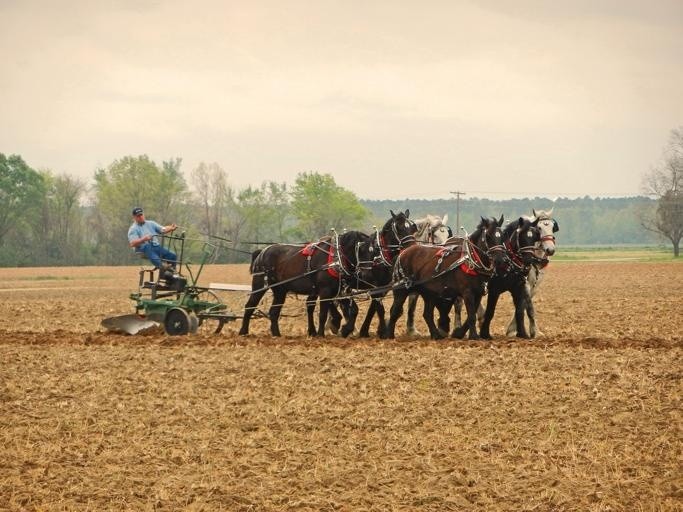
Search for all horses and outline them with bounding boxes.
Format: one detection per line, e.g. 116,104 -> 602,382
237,231 -> 377,338
413,213 -> 450,244
423,216 -> 541,339
327,209 -> 417,338
386,213 -> 513,340
455,206 -> 559,339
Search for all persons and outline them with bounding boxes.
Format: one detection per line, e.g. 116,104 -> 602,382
127,208 -> 177,271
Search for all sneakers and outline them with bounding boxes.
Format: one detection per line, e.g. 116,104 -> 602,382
161,262 -> 172,270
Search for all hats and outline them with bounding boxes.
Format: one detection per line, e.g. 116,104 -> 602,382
132,207 -> 143,216
